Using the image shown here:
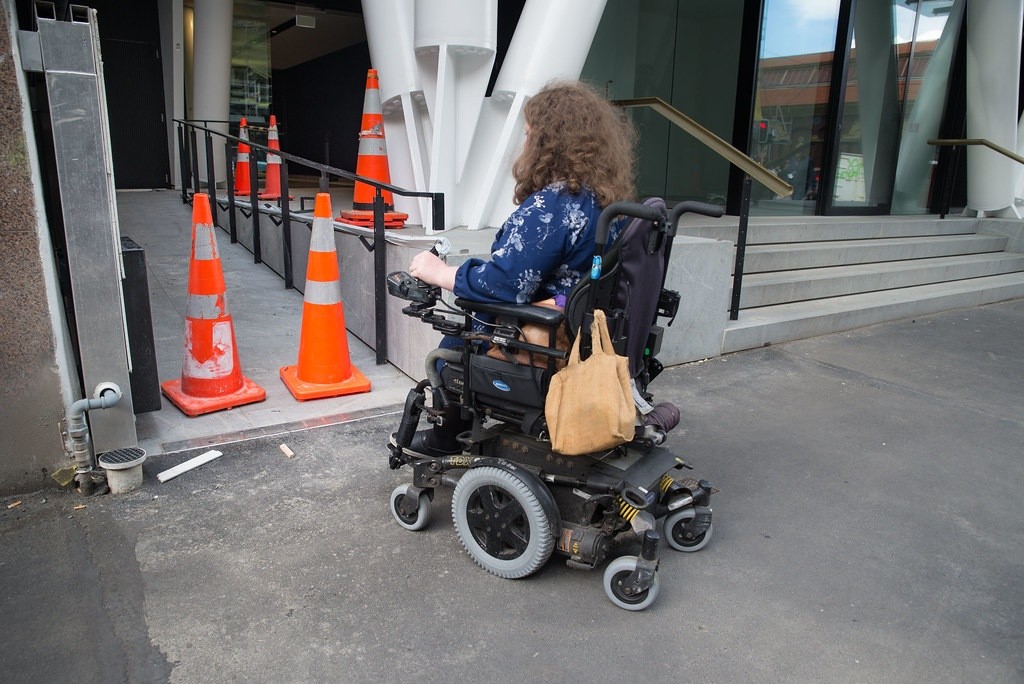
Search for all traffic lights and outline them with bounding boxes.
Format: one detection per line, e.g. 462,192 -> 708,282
758,120 -> 769,142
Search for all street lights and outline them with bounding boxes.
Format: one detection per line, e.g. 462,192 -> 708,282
605,79 -> 614,100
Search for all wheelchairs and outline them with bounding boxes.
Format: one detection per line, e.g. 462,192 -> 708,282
381,197 -> 729,612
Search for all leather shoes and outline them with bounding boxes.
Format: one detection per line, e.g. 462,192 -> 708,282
390,428 -> 464,460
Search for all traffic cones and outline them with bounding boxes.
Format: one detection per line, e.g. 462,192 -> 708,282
158,193 -> 267,418
230,117 -> 256,194
255,114 -> 295,201
278,193 -> 373,402
332,68 -> 409,231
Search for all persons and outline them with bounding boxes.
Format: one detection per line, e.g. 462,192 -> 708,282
389,83 -> 640,459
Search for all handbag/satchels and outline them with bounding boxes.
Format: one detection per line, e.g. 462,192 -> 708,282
545,309 -> 636,456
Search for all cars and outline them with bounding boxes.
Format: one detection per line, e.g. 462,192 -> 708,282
805,167 -> 821,200
748,138 -> 863,202
